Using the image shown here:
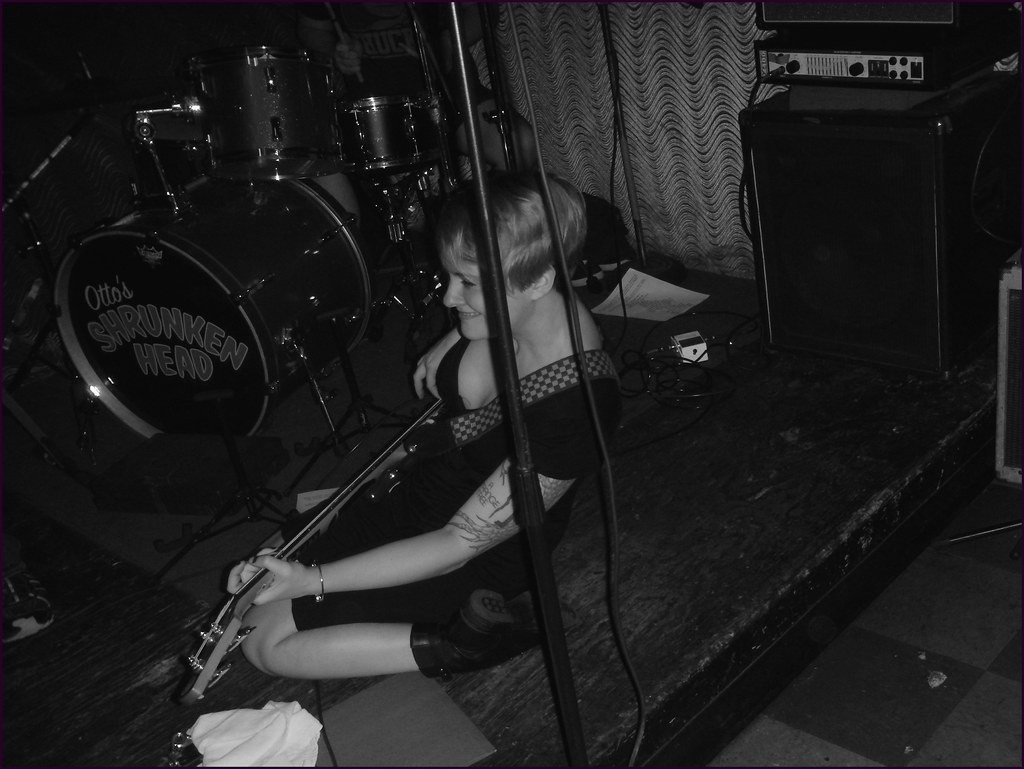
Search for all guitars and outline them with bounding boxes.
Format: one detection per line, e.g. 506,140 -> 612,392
173,396 -> 448,705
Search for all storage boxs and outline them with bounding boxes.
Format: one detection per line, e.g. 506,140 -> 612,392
995,247 -> 1024,485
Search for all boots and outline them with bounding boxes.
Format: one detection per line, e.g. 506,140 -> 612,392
409,587 -> 578,680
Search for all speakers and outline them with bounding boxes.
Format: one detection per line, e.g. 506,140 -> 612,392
738,59 -> 1024,383
997,244 -> 1021,485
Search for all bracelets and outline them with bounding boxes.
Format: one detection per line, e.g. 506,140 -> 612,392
309,561 -> 325,602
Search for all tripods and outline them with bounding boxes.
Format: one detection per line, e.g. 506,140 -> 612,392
1,107 -> 458,589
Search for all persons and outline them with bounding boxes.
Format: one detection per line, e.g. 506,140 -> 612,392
227,169 -> 625,680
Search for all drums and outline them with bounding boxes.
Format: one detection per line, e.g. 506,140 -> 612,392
51,170 -> 373,436
329,93 -> 420,178
184,46 -> 339,181
